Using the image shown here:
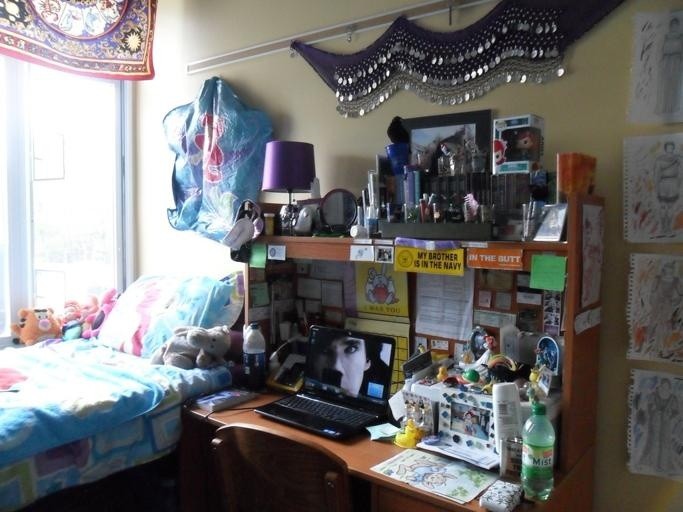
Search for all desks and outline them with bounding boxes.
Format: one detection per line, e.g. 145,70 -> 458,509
182,381 -> 595,512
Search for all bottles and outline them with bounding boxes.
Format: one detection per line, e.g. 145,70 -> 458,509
242,322 -> 266,391
521,402 -> 556,500
262,212 -> 275,236
491,380 -> 523,454
366,206 -> 378,239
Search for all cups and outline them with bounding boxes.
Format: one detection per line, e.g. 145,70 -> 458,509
385,142 -> 410,174
386,203 -> 498,226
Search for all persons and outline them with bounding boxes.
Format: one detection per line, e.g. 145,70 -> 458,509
312,329 -> 383,399
463,410 -> 487,440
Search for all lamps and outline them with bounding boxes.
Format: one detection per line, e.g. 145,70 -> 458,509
258,140 -> 314,233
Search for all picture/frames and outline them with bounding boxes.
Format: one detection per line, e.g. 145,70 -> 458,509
399,109 -> 490,191
436,388 -> 499,457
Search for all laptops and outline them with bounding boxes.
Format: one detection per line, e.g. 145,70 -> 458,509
253,324 -> 397,441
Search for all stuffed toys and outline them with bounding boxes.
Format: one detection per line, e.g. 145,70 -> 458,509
10,288 -> 120,346
150,325 -> 234,370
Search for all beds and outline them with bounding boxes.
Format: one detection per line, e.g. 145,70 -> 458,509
0,334 -> 246,512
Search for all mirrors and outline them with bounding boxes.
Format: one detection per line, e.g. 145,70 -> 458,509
318,190 -> 358,235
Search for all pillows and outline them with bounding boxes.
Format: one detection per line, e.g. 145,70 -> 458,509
98,270 -> 246,361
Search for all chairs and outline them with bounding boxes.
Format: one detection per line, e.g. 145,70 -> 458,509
208,424 -> 351,512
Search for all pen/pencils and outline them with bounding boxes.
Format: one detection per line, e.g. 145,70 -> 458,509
303,311 -> 320,324
